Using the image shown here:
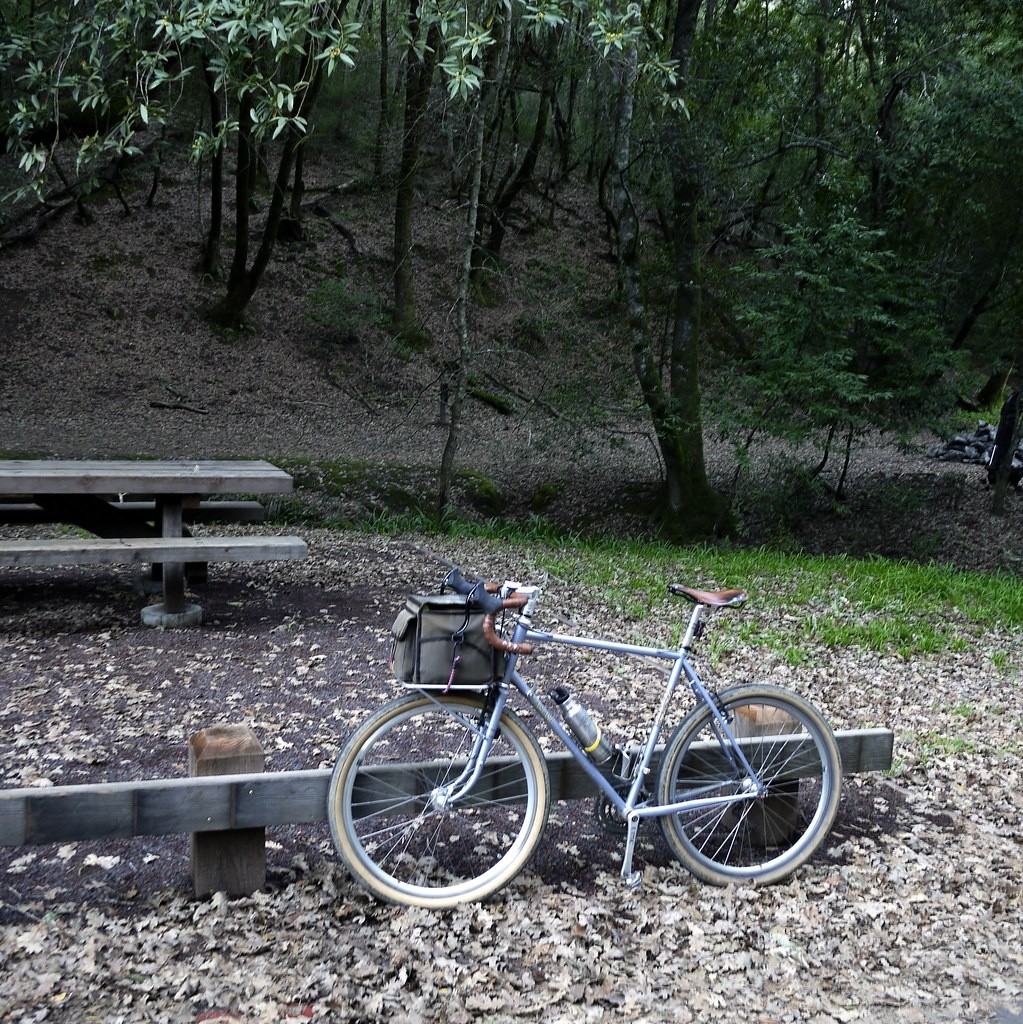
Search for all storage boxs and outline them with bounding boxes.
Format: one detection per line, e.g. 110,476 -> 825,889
389,595 -> 495,684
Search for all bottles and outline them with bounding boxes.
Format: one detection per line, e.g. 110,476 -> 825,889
546,686 -> 613,765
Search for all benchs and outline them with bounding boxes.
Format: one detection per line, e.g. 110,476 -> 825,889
0,502 -> 263,525
0,535 -> 307,567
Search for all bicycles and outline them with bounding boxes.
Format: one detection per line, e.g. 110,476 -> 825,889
326,568 -> 845,909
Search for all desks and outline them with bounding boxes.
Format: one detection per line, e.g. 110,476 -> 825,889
0,460 -> 308,627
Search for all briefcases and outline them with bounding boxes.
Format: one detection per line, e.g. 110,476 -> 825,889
390,593 -> 505,685
